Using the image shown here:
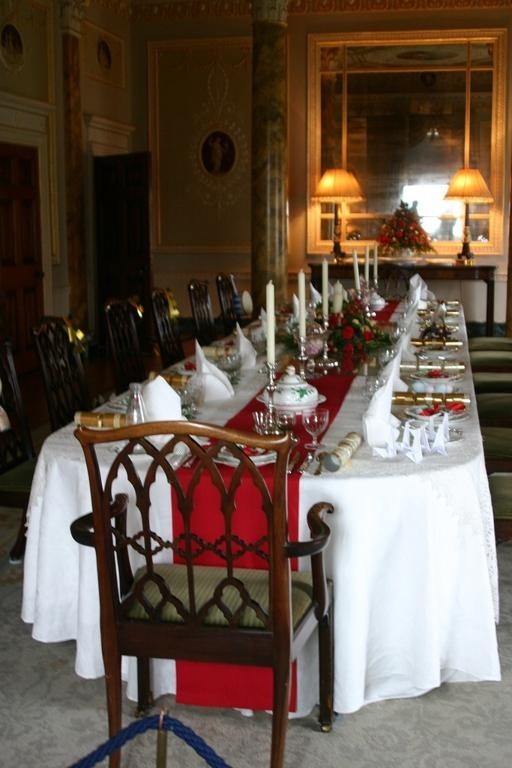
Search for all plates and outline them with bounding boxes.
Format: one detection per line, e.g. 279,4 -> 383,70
85,283 -> 470,470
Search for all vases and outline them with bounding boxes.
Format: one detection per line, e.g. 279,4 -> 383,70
396,247 -> 416,260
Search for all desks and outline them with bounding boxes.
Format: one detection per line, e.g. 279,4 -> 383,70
308,261 -> 497,336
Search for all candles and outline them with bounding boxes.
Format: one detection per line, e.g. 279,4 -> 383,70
352,248 -> 360,291
364,245 -> 370,284
374,242 -> 378,281
322,259 -> 329,319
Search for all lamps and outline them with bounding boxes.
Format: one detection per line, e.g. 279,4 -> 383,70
311,169 -> 368,258
445,169 -> 494,259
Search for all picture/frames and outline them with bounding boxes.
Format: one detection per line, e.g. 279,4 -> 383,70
147,32 -> 291,257
80,18 -> 128,92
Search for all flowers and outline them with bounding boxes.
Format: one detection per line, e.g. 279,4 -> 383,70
377,201 -> 437,258
